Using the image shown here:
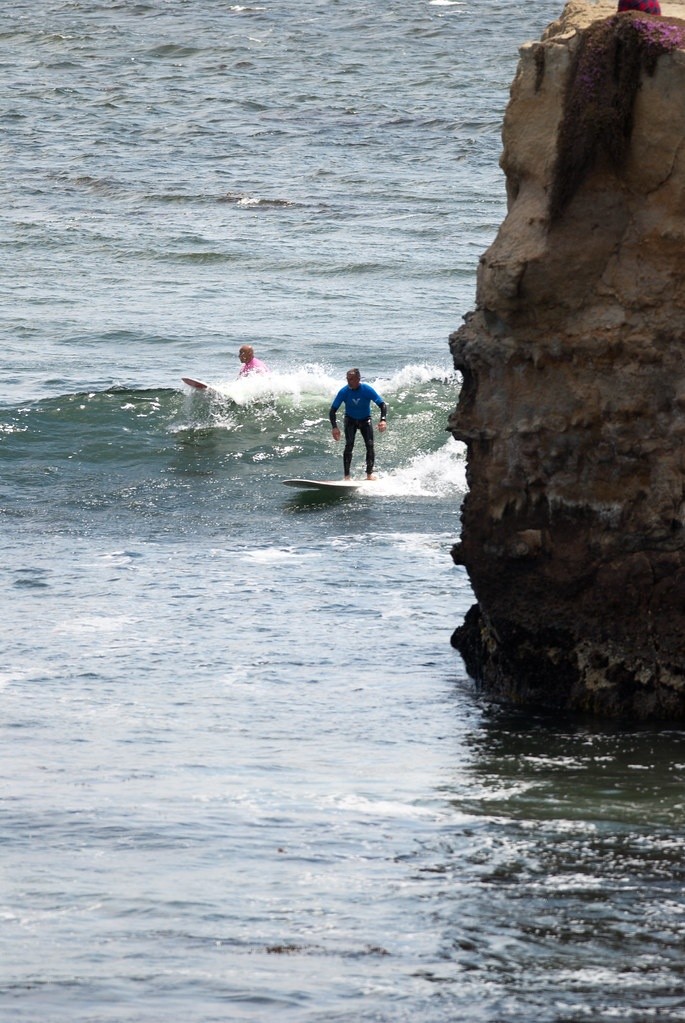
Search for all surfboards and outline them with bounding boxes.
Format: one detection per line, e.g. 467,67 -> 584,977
282,478 -> 376,489
181,377 -> 211,392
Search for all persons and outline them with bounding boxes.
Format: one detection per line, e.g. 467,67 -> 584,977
617,0 -> 661,16
237,345 -> 268,377
328,369 -> 388,481
